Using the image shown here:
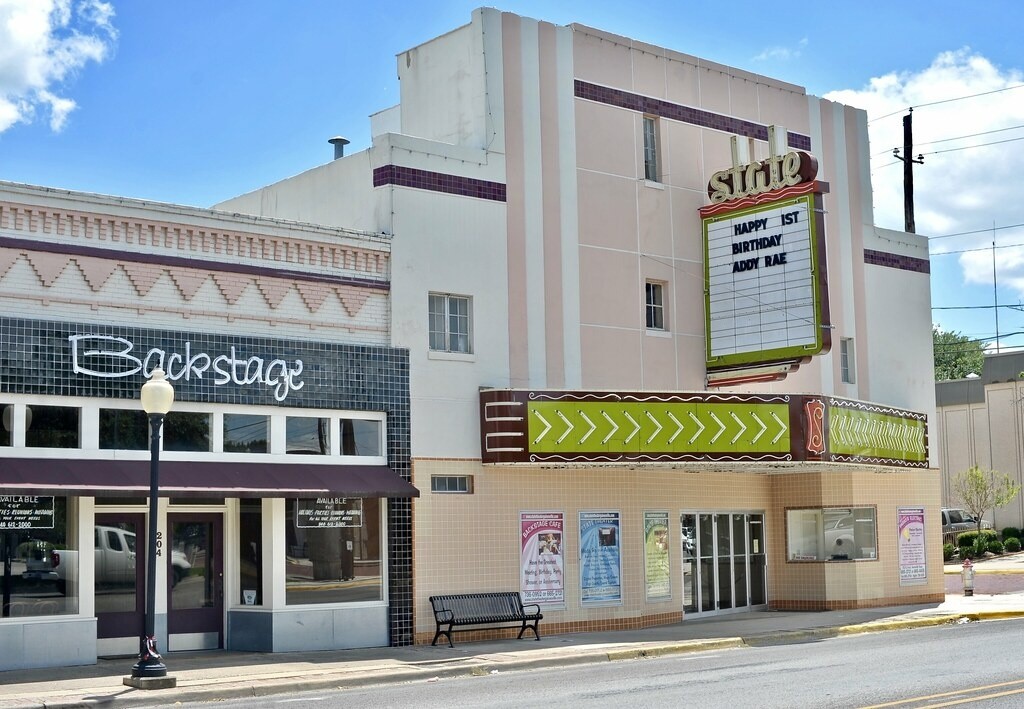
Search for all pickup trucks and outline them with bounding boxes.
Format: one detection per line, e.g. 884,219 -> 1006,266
23,526 -> 191,595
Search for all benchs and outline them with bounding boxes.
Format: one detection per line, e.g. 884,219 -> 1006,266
429,592 -> 543,649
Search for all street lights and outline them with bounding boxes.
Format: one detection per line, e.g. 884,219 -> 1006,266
131,359 -> 175,676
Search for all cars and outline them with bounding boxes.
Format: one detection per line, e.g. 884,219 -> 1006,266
686,527 -> 696,555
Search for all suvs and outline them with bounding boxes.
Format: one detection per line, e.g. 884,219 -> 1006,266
941,507 -> 992,544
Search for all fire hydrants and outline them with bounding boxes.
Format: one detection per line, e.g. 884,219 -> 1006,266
963,558 -> 975,595
